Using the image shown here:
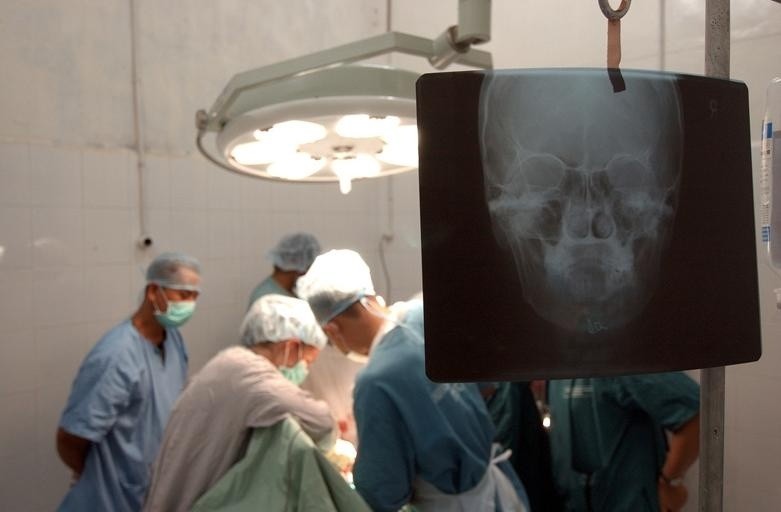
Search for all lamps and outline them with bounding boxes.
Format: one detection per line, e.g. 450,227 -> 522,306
196,0 -> 493,195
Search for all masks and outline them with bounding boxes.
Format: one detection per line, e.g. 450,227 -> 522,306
153,287 -> 196,328
278,341 -> 309,386
327,323 -> 368,363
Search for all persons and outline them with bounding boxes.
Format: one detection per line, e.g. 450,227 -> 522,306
142,293 -> 338,511
543,368 -> 703,511
244,232 -> 324,321
300,249 -> 535,511
55,250 -> 203,512
479,374 -> 560,511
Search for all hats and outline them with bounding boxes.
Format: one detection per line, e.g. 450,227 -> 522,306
293,249 -> 376,327
272,232 -> 321,274
238,294 -> 328,351
145,252 -> 202,292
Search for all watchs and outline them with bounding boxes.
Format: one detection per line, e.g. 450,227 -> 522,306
657,468 -> 685,489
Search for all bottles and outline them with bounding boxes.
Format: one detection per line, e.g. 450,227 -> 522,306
760,75 -> 780,307
